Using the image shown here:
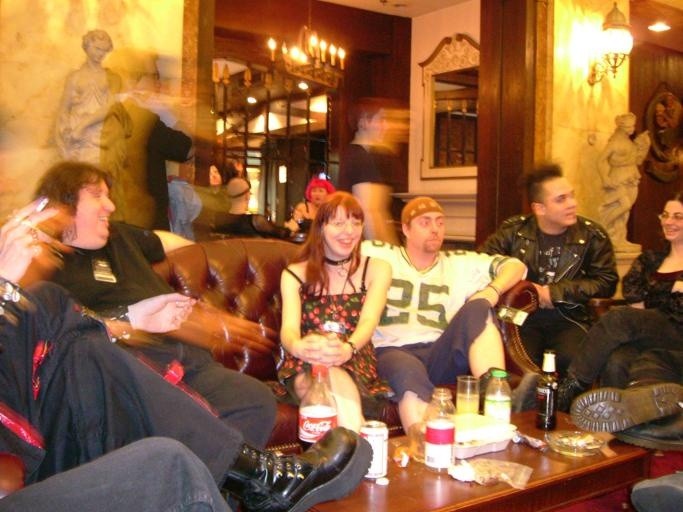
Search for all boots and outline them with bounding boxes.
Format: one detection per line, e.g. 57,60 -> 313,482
222,426 -> 374,512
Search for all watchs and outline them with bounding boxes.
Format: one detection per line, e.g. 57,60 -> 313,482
487,284 -> 501,299
346,341 -> 357,360
475,163 -> 619,358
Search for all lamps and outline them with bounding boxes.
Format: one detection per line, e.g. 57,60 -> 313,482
589,2 -> 635,87
267,0 -> 346,91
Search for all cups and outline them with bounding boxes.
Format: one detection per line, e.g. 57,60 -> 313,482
455,377 -> 482,414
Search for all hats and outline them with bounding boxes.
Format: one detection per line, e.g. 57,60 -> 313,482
305,176 -> 336,201
399,194 -> 445,227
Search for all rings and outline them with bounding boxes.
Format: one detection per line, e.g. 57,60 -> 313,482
28,228 -> 39,244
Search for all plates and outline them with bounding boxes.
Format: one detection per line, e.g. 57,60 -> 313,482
548,432 -> 606,457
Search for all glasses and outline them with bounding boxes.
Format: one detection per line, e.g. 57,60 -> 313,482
657,213 -> 682,221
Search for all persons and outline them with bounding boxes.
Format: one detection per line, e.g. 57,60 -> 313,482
568,348 -> 682,453
356,194 -> 529,438
31,160 -> 278,450
275,191 -> 393,437
598,112 -> 651,253
555,186 -> 682,451
283,179 -> 337,233
337,94 -> 411,246
54,29 -> 136,224
208,177 -> 299,239
0,197 -> 375,510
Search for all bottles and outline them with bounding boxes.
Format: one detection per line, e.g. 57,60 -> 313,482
536,350 -> 558,431
298,366 -> 340,453
424,388 -> 454,472
358,420 -> 389,481
485,371 -> 512,431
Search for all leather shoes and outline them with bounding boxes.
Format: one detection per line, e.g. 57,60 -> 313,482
613,414 -> 682,452
570,380 -> 683,433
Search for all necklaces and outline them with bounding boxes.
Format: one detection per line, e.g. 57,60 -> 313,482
324,256 -> 351,278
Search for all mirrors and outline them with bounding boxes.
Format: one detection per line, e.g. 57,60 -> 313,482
418,32 -> 479,180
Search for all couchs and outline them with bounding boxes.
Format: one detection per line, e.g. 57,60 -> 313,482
146,238 -> 559,512
